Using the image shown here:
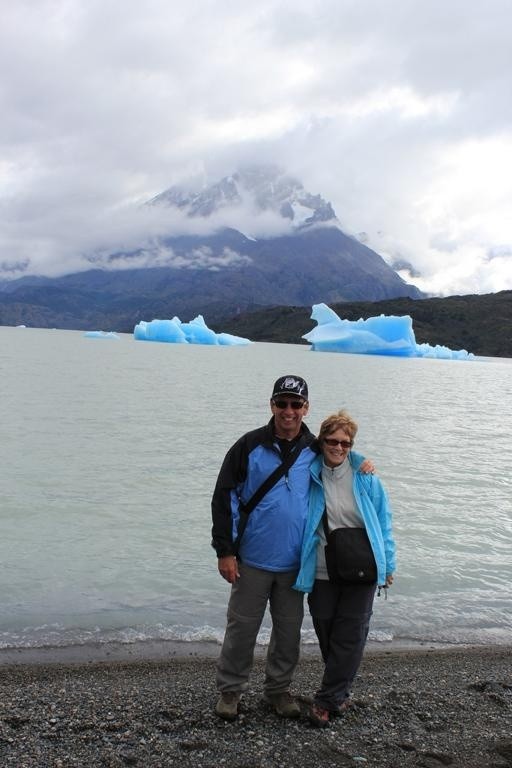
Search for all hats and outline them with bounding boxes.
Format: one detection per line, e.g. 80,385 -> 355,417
272,375 -> 309,401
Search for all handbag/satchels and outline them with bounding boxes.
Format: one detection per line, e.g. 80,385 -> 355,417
324,526 -> 378,586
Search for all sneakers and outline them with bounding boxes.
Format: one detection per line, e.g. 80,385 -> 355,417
214,695 -> 238,720
262,690 -> 301,718
311,699 -> 349,727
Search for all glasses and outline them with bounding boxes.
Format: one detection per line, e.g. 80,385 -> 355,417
272,399 -> 307,409
324,438 -> 351,448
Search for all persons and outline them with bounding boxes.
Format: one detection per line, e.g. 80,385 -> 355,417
207,372 -> 377,721
291,407 -> 398,726
